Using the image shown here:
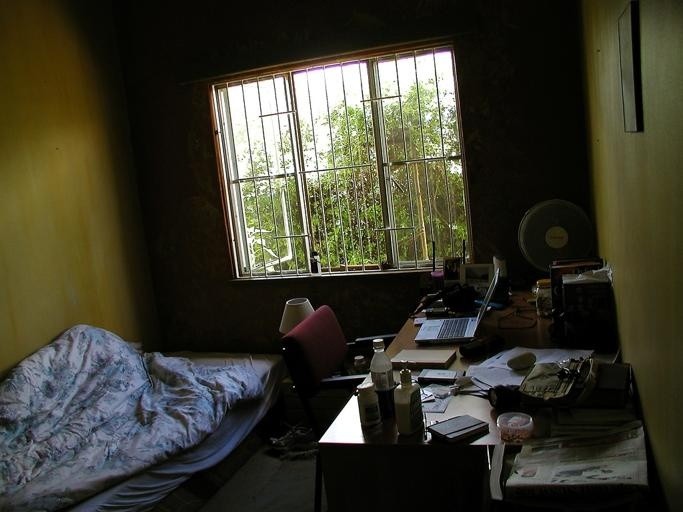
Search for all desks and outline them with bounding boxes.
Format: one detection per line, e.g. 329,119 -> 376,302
317,284 -> 648,511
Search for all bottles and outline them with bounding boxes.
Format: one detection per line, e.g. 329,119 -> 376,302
370,339 -> 396,422
536,279 -> 552,320
394,361 -> 424,436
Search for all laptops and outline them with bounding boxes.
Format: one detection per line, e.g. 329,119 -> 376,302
414,267 -> 500,345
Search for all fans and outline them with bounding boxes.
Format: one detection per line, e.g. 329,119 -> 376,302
519,198 -> 594,271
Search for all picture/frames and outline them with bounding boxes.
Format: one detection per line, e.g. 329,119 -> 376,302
617,1 -> 644,134
443,257 -> 494,288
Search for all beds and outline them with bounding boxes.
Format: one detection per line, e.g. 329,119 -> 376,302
0,350 -> 285,511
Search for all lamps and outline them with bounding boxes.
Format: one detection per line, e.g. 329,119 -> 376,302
279,298 -> 316,334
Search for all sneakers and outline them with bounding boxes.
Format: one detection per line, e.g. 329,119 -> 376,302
272,424 -> 314,451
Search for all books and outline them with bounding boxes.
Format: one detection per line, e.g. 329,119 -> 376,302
535,259 -> 610,332
390,349 -> 456,369
418,368 -> 457,384
429,415 -> 489,445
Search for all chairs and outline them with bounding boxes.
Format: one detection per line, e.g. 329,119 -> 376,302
280,305 -> 396,432
